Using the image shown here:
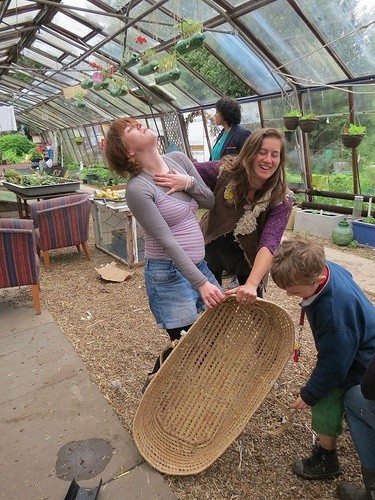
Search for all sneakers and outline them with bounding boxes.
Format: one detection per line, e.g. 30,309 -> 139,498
292,436 -> 340,479
337,484 -> 374,500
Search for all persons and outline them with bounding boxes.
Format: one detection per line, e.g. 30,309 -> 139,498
336,357 -> 375,500
270,234 -> 375,479
192,128 -> 292,359
104,118 -> 226,393
212,97 -> 252,290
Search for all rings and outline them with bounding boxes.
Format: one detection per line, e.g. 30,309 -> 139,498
242,297 -> 246,301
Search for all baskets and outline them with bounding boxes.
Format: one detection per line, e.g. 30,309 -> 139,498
132,292 -> 295,477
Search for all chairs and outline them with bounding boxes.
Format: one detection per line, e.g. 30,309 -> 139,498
30,194 -> 93,271
0,218 -> 41,315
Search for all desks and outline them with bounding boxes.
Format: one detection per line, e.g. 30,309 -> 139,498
17,191 -> 76,219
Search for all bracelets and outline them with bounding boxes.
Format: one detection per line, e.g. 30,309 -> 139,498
184,174 -> 188,191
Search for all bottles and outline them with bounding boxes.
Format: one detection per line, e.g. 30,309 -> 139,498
331,221 -> 353,246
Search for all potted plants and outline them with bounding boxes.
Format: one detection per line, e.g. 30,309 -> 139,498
76,50 -> 179,107
286,208 -> 375,247
298,113 -> 318,132
281,108 -> 301,130
3,137 -> 123,195
176,19 -> 205,55
341,123 -> 366,147
